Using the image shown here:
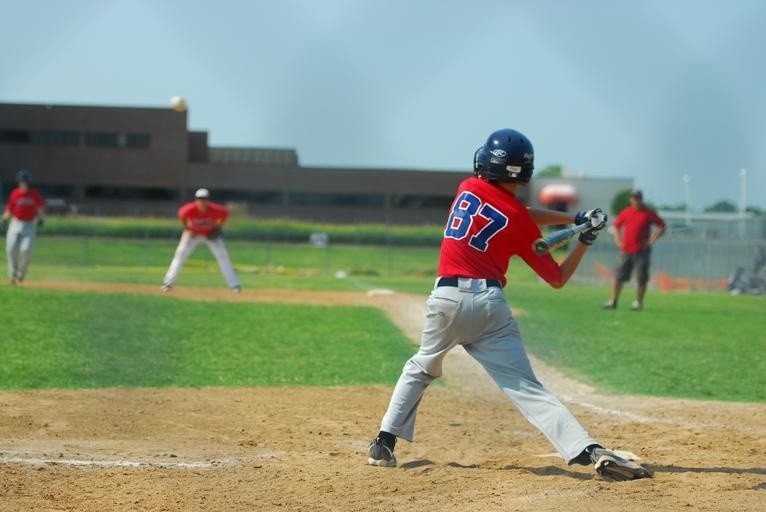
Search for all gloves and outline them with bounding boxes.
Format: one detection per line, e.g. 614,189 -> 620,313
575,207 -> 607,245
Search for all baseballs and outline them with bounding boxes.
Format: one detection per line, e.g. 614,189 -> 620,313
172,96 -> 189,113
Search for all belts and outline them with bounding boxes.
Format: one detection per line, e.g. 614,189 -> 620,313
437,276 -> 503,288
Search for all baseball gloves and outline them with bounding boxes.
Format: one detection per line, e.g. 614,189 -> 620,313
206,228 -> 223,239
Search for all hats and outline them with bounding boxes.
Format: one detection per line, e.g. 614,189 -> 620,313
629,190 -> 642,198
196,188 -> 210,200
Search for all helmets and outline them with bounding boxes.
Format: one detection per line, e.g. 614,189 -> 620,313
16,170 -> 32,186
474,129 -> 534,183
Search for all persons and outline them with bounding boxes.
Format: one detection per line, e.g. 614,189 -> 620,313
601,188 -> 665,310
367,128 -> 652,482
161,188 -> 240,292
2,170 -> 46,284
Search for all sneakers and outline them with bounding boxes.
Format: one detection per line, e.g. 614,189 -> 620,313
585,447 -> 653,481
368,438 -> 397,467
601,300 -> 642,310
232,285 -> 241,292
162,282 -> 171,294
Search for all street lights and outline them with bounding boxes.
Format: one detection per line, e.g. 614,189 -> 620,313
740,167 -> 748,229
683,173 -> 696,225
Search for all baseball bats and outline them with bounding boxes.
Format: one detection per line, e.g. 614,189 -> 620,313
532,221 -> 591,255
